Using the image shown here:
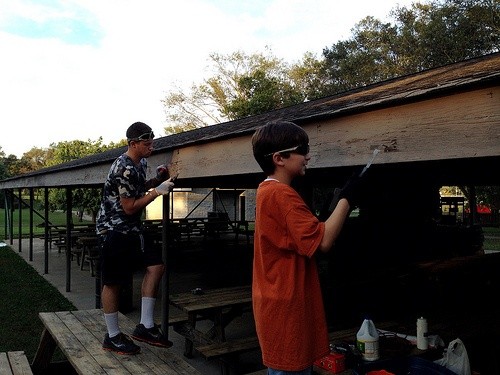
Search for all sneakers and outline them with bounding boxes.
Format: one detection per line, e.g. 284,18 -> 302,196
102,332 -> 141,354
131,324 -> 173,348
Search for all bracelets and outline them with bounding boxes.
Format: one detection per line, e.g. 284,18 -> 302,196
149,192 -> 154,201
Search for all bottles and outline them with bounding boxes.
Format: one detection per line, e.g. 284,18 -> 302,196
356,312 -> 380,362
416,316 -> 429,351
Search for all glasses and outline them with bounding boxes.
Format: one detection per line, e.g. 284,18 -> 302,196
265,144 -> 311,158
128,131 -> 155,142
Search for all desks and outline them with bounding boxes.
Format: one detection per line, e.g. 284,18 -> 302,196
176,220 -> 255,242
142,217 -> 220,226
311,319 -> 460,375
169,284 -> 252,375
75,237 -> 98,277
31,308 -> 201,375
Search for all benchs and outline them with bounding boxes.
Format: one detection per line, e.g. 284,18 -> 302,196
40,236 -> 100,260
0,350 -> 34,375
194,336 -> 260,360
154,306 -> 251,328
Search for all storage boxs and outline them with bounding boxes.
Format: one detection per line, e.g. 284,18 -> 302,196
314,352 -> 345,373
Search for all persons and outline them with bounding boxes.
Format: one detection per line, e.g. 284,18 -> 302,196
252,121 -> 367,375
97,122 -> 175,354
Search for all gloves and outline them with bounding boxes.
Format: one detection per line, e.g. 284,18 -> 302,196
334,171 -> 362,202
155,175 -> 176,195
